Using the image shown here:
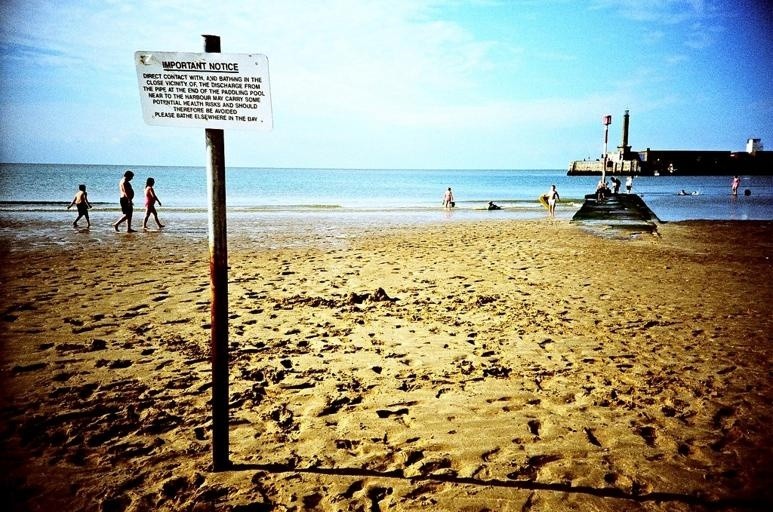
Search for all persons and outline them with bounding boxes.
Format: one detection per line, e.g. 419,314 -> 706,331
112,171 -> 137,233
67,184 -> 94,228
486,199 -> 501,210
443,186 -> 453,209
545,185 -> 560,216
142,178 -> 165,229
596,174 -> 751,199
